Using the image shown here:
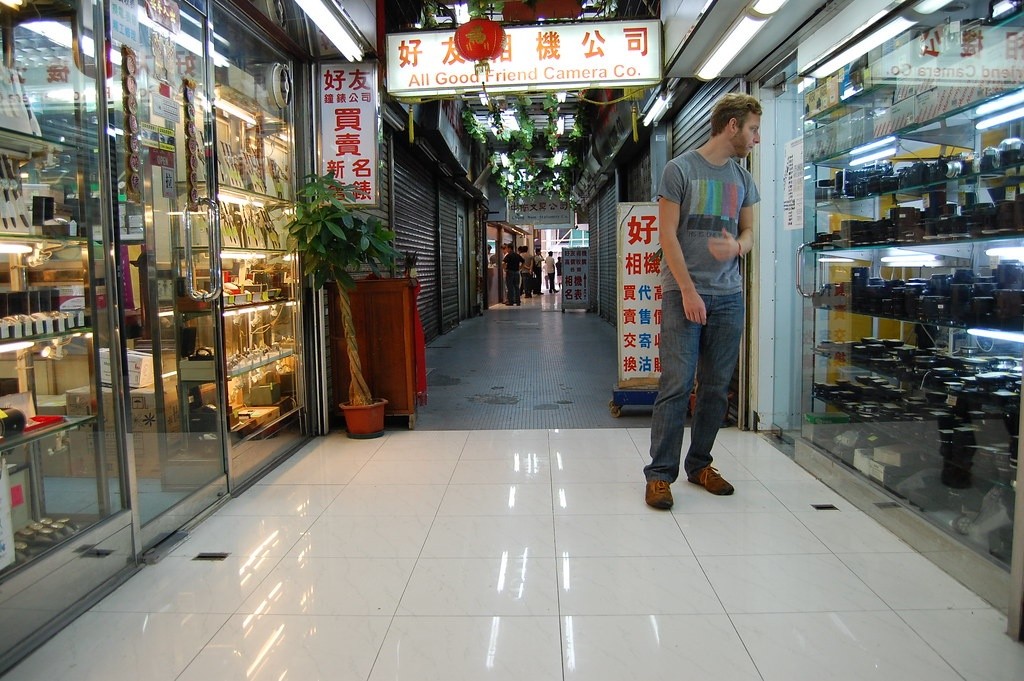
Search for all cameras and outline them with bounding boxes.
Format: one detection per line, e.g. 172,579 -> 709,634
830,138 -> 1024,200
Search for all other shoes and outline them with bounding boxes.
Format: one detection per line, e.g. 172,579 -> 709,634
505,303 -> 513,306
537,292 -> 544,295
517,302 -> 520,306
524,296 -> 532,298
554,291 -> 559,293
549,290 -> 552,293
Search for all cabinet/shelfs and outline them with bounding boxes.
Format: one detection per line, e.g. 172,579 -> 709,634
174,85 -> 307,456
323,278 -> 419,428
0,125 -> 111,580
809,10 -> 1024,571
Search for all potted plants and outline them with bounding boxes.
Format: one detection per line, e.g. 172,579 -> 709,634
286,174 -> 406,440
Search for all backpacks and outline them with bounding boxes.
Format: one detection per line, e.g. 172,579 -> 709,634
533,257 -> 539,272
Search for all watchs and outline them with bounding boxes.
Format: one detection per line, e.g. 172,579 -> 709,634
16,516 -> 78,559
218,140 -> 289,200
228,341 -> 283,368
196,347 -> 212,356
220,200 -> 281,250
0,308 -> 74,325
0,155 -> 30,230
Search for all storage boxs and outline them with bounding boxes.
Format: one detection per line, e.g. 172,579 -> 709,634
238,406 -> 280,428
66,378 -> 181,477
98,347 -> 164,389
250,382 -> 281,406
35,392 -> 70,477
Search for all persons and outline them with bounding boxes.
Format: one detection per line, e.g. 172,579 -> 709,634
488,243 -> 562,306
643,93 -> 760,512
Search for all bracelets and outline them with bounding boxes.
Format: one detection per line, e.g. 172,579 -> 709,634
736,241 -> 742,256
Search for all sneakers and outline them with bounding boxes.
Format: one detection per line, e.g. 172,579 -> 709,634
686,464 -> 735,497
644,477 -> 675,511
